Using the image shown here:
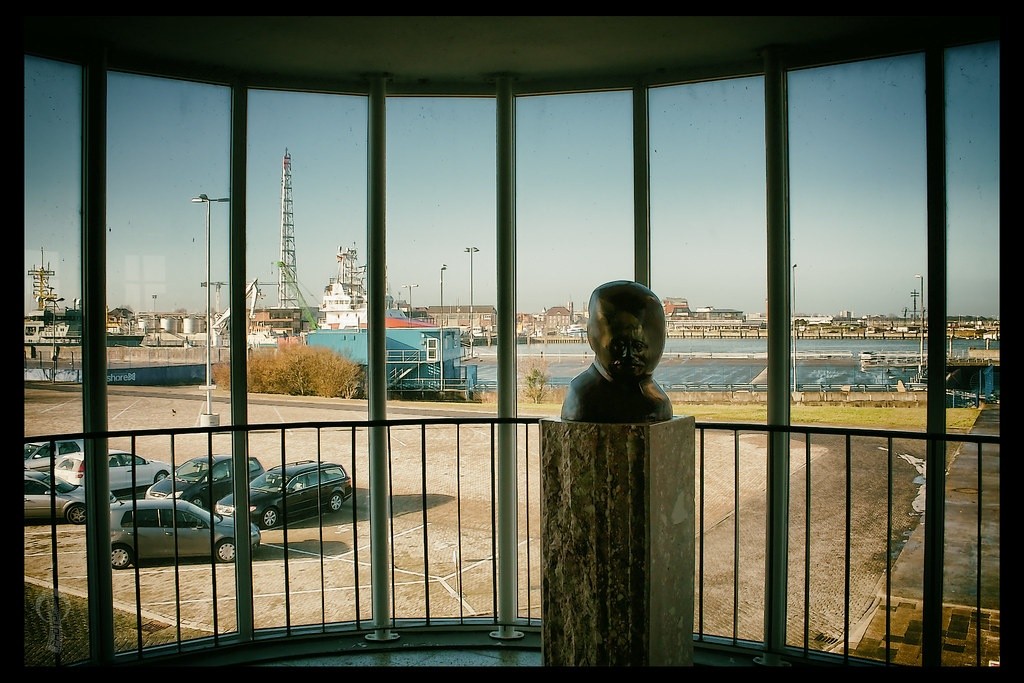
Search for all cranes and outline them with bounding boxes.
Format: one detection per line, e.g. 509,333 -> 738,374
200,280 -> 279,316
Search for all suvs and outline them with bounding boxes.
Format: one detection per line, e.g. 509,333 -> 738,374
144,454 -> 266,509
55,449 -> 172,496
240,460 -> 353,529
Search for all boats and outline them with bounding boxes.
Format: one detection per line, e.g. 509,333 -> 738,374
24,245 -> 146,351
302,238 -> 478,401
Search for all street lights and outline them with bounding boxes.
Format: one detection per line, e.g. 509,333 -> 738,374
440,266 -> 445,392
44,297 -> 65,384
915,273 -> 924,369
402,283 -> 417,328
464,247 -> 480,358
152,294 -> 157,338
191,192 -> 231,429
792,263 -> 797,392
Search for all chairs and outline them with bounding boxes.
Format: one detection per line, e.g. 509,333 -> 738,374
109,457 -> 118,467
293,481 -> 303,490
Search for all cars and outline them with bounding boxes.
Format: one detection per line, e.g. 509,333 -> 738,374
111,499 -> 263,570
25,440 -> 85,469
24,472 -> 117,525
